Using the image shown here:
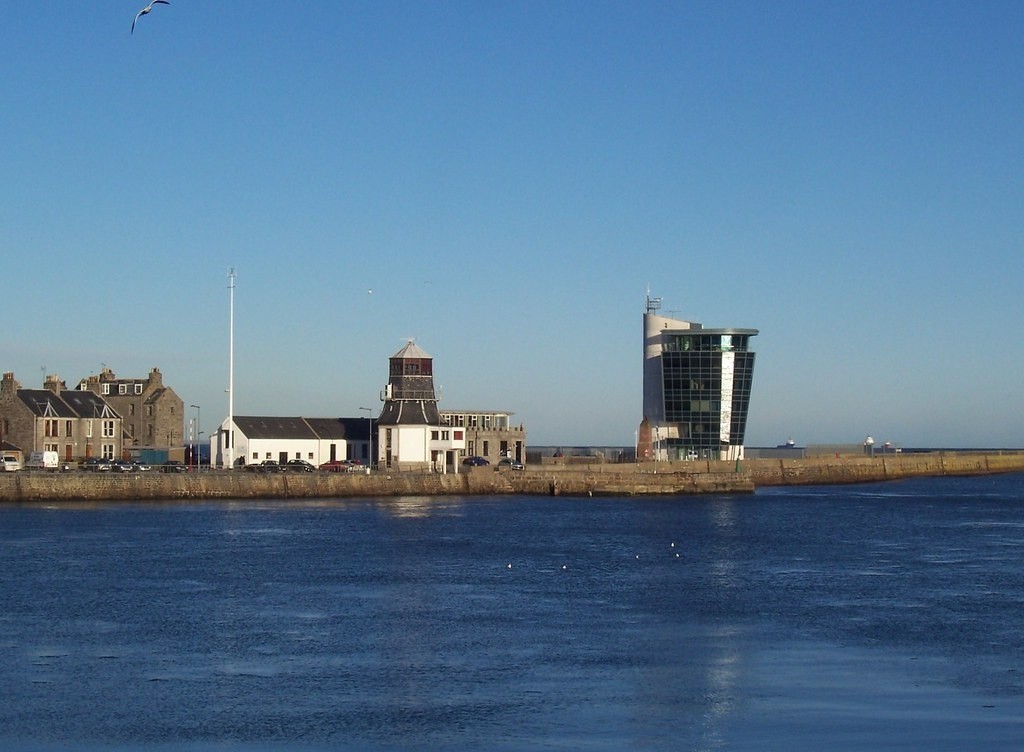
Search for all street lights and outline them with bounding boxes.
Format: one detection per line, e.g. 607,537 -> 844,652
190,405 -> 200,473
359,407 -> 371,469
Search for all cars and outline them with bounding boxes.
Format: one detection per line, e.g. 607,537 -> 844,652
112,460 -> 134,473
284,459 -> 316,472
498,458 -> 526,471
88,458 -> 113,472
243,460 -> 282,473
134,461 -> 152,472
0,455 -> 21,472
161,461 -> 189,473
463,457 -> 490,467
319,461 -> 349,472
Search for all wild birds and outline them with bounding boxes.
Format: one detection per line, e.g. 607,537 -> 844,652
128,0 -> 171,36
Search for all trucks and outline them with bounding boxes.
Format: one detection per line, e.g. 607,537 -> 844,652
25,451 -> 58,472
